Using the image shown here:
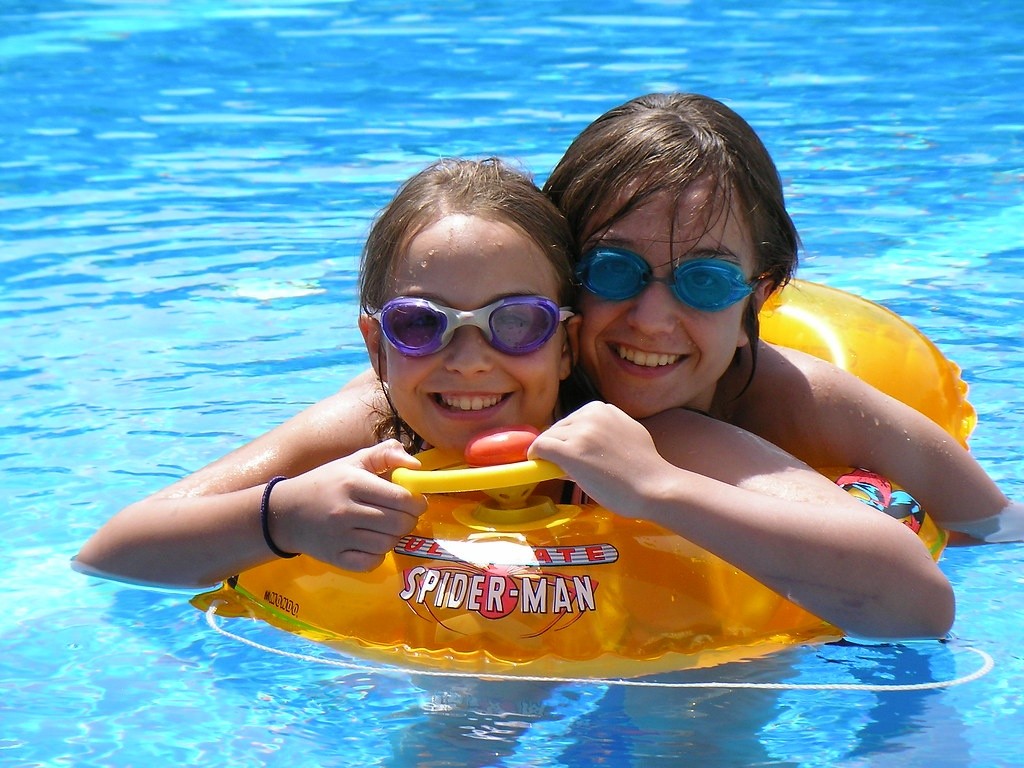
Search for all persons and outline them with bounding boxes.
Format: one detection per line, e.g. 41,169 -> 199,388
73,157 -> 957,642
341,92 -> 1009,524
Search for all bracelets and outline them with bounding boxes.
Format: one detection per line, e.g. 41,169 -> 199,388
259,474 -> 302,559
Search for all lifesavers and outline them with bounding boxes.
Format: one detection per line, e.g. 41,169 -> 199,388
188,278 -> 975,688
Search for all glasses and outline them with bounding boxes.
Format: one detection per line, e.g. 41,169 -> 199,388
571,248 -> 778,312
364,297 -> 579,355
